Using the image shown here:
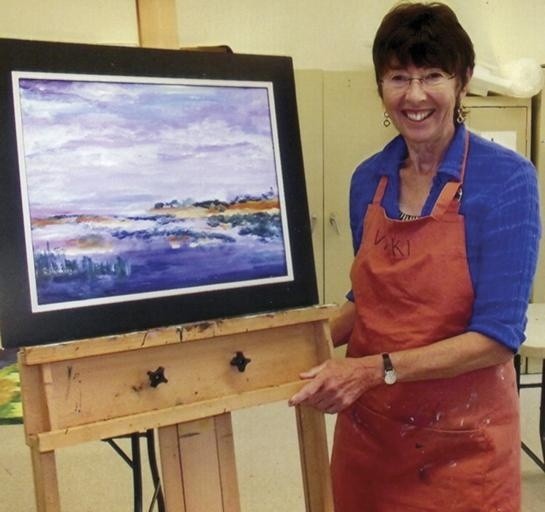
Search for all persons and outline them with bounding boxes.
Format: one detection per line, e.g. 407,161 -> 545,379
288,0 -> 545,512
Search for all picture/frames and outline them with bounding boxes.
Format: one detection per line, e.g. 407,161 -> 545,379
0,36 -> 321,349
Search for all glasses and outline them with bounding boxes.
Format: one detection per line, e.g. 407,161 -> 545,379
379,70 -> 457,87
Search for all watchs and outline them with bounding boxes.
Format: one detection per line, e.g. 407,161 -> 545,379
382,352 -> 397,385
